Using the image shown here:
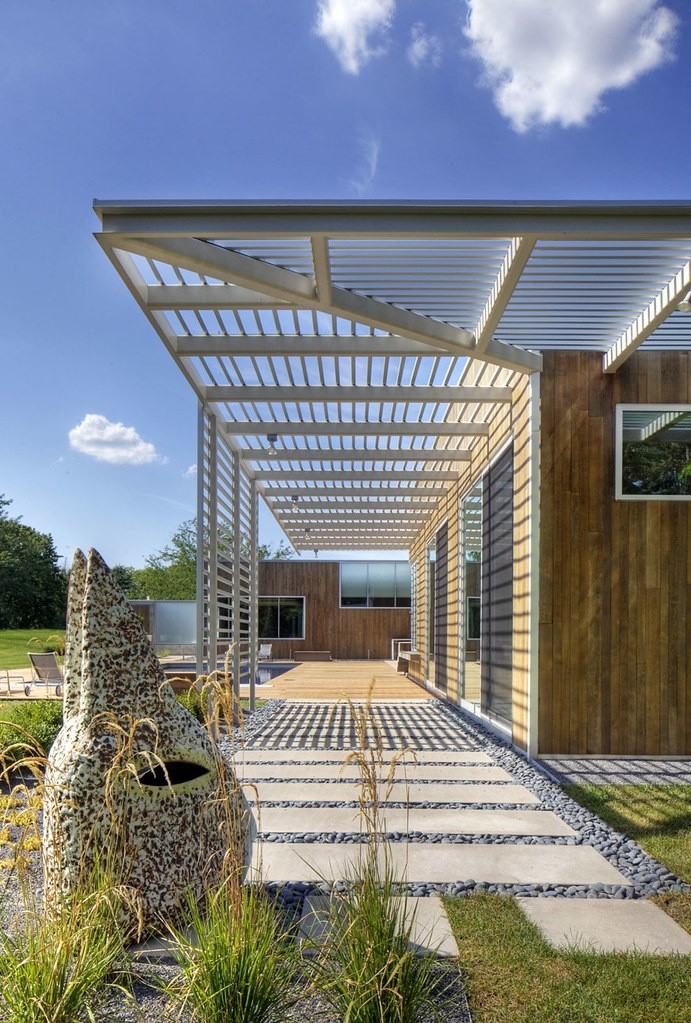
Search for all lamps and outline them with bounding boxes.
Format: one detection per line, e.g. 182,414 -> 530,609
267,434 -> 277,456
305,528 -> 311,540
291,496 -> 298,510
314,549 -> 319,558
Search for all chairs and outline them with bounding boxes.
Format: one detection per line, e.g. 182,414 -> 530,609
0,670 -> 25,696
24,652 -> 64,696
258,644 -> 273,662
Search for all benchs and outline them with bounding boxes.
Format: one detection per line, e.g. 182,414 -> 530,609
294,650 -> 331,661
164,672 -> 233,697
